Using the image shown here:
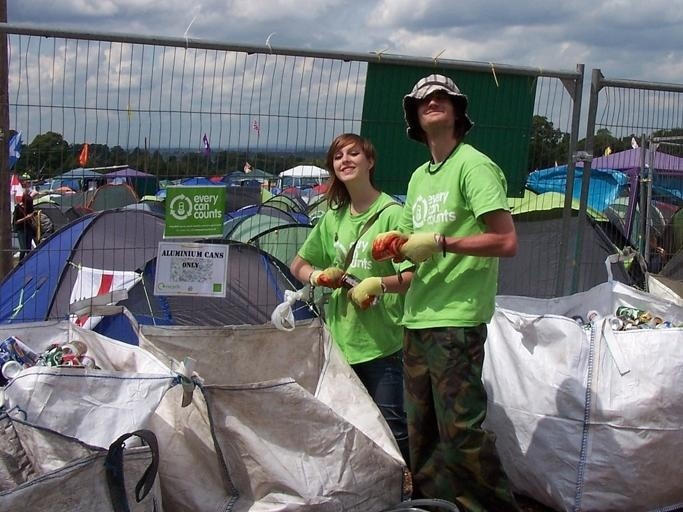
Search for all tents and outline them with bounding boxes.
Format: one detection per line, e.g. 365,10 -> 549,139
2,209 -> 322,345
37,148 -> 682,322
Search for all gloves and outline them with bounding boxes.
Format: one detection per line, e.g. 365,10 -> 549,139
347,277 -> 385,309
309,266 -> 344,290
401,232 -> 442,264
372,230 -> 404,264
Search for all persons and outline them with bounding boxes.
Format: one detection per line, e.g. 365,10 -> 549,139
373,75 -> 518,512
289,133 -> 415,472
11,191 -> 39,262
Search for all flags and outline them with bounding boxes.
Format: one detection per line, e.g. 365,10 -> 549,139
78,142 -> 87,167
202,133 -> 211,159
10,175 -> 24,203
126,101 -> 134,121
6,132 -> 20,172
252,119 -> 261,133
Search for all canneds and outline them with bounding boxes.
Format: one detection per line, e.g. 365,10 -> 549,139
573,304 -> 672,333
341,273 -> 378,306
372,239 -> 406,263
0,335 -> 97,380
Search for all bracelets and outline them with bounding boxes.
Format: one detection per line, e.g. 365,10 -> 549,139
441,234 -> 446,257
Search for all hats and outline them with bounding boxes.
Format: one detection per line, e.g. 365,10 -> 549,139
403,74 -> 475,145
